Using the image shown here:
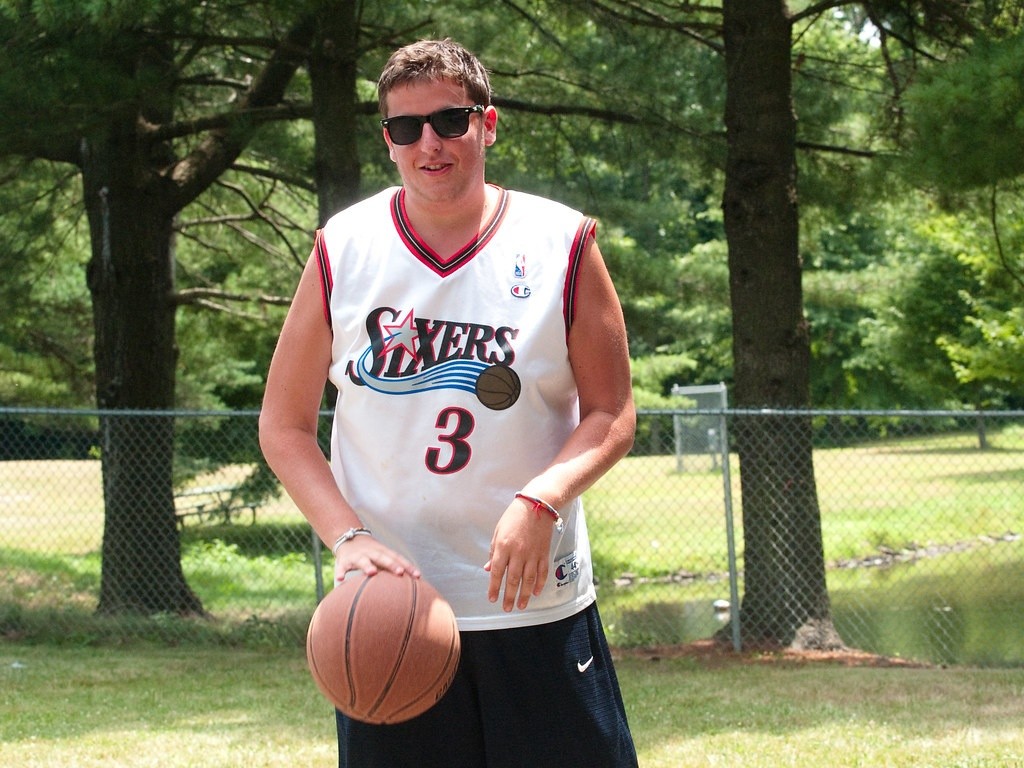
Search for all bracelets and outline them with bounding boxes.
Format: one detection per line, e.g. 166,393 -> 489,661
514,492 -> 564,533
333,527 -> 372,551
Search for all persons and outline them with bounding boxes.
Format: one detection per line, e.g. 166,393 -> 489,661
258,36 -> 637,768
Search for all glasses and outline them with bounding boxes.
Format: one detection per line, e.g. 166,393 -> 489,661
379,105 -> 485,145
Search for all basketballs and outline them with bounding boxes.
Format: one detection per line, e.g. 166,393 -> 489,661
306,570 -> 462,726
